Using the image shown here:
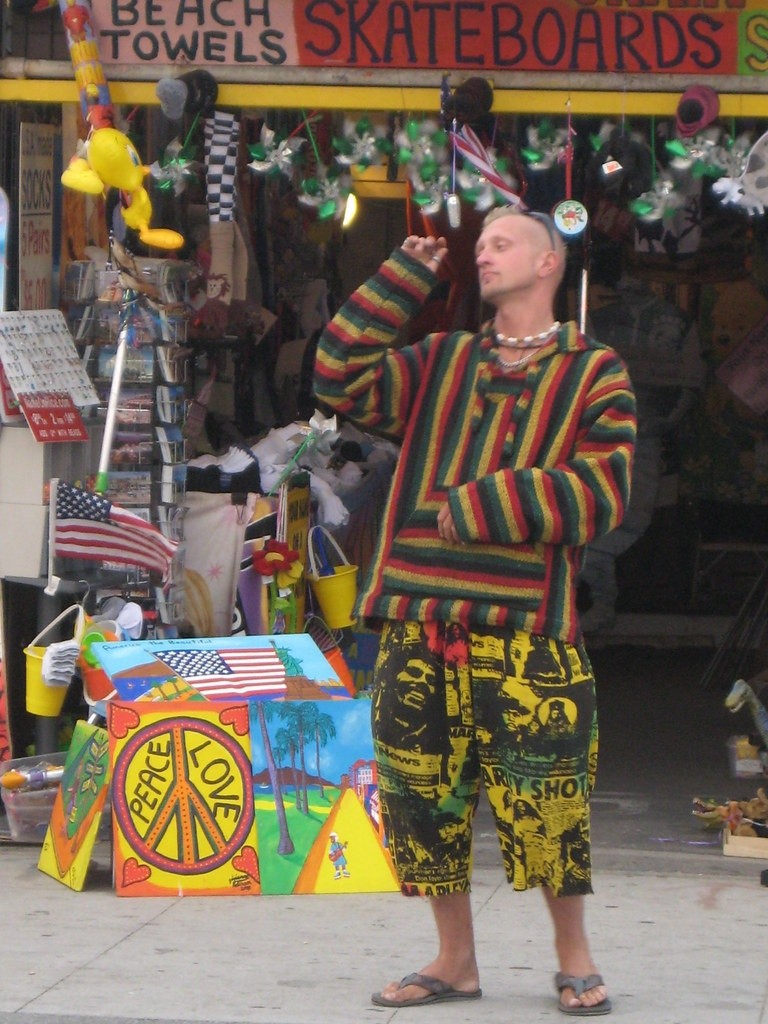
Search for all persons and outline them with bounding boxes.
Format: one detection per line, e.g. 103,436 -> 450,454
313,205 -> 637,1017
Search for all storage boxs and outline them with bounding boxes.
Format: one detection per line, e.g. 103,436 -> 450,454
0,750 -> 113,843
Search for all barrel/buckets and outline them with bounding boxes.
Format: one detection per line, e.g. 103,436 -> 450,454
307,524 -> 360,629
23,603 -> 146,717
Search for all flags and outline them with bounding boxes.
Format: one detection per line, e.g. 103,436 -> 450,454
54,482 -> 178,592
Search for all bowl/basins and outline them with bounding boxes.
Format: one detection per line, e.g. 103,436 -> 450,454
725,735 -> 768,779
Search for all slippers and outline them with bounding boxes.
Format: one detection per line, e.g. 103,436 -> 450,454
554,970 -> 613,1017
371,972 -> 484,1008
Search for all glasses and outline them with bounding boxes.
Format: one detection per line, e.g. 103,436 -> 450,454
525,211 -> 556,251
